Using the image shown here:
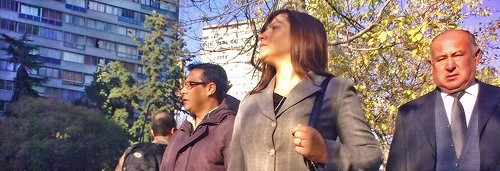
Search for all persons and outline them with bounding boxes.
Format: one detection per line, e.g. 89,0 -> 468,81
385,29 -> 500,171
223,10 -> 385,171
158,63 -> 235,171
115,111 -> 177,171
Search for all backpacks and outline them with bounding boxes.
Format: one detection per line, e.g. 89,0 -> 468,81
121,142 -> 167,171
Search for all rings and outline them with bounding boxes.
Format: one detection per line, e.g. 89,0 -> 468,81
298,139 -> 302,146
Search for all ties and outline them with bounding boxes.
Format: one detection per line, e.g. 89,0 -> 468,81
447,90 -> 467,161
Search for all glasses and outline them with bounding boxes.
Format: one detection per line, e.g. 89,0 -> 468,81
185,81 -> 206,90
